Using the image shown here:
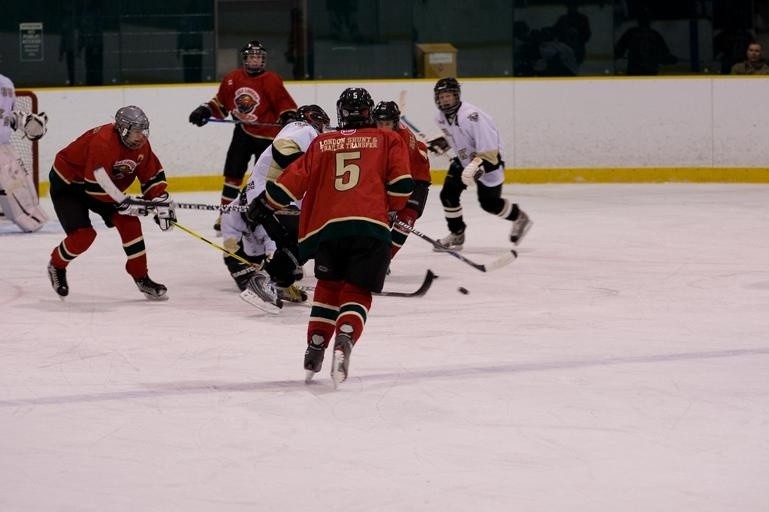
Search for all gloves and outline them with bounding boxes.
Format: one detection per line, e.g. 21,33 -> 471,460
189,104 -> 212,126
425,136 -> 451,158
114,195 -> 154,217
246,191 -> 272,232
280,110 -> 295,127
461,156 -> 486,186
151,195 -> 178,231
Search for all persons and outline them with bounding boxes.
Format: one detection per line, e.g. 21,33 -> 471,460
0,73 -> 48,233
188,41 -> 530,382
47,105 -> 176,297
730,42 -> 769,74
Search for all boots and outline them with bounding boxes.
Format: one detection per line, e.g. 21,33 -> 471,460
47,259 -> 68,295
132,274 -> 167,297
433,229 -> 464,249
246,258 -> 307,309
304,330 -> 325,371
511,210 -> 528,243
331,322 -> 352,381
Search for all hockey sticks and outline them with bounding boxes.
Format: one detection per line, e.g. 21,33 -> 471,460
398,91 -> 465,173
299,270 -> 432,297
150,209 -> 266,271
393,218 -> 517,272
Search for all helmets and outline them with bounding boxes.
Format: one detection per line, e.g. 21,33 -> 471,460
242,41 -> 267,76
374,101 -> 401,133
434,78 -> 461,114
336,89 -> 374,129
296,105 -> 330,133
115,105 -> 150,149
